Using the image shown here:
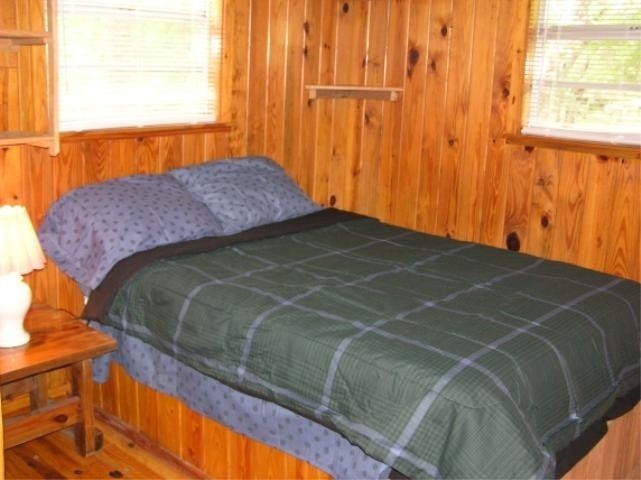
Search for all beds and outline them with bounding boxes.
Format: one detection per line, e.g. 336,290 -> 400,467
60,156 -> 641,480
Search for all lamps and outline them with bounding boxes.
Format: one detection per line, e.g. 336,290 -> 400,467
2,205 -> 47,348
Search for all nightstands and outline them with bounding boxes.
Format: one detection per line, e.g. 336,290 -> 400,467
0,307 -> 118,457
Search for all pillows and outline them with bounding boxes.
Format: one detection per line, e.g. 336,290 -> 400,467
172,157 -> 316,231
37,174 -> 220,291
